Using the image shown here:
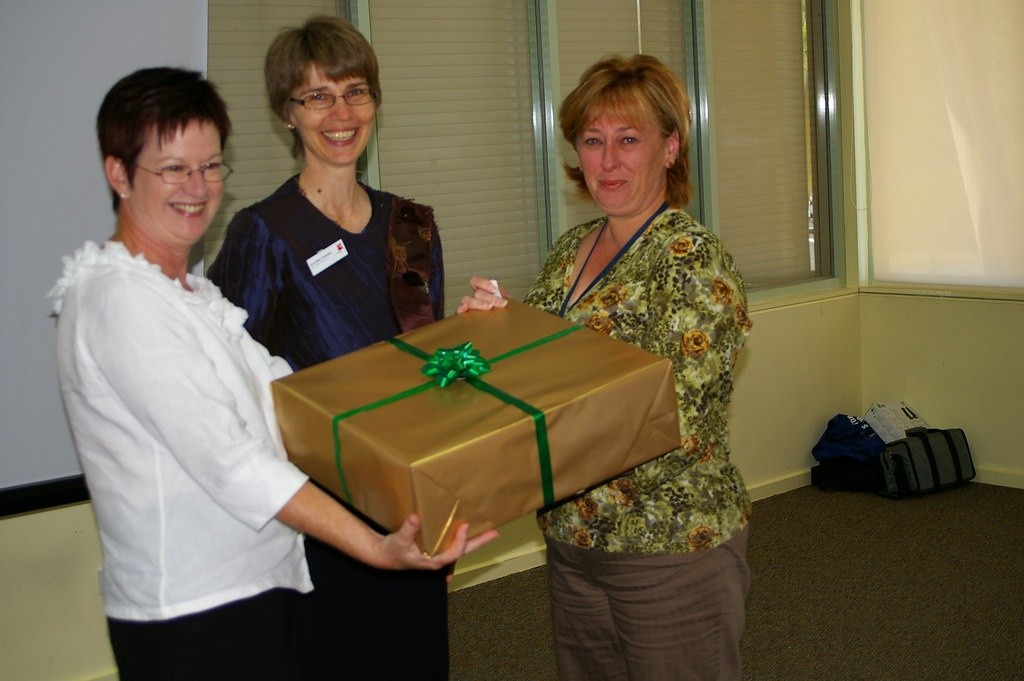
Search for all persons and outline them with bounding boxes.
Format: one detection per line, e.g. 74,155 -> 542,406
44,65 -> 500,681
203,18 -> 457,680
453,55 -> 757,681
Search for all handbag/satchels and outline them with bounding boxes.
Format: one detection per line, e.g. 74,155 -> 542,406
881,426 -> 976,494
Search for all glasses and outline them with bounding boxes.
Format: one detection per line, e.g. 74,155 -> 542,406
287,83 -> 375,110
125,160 -> 234,184
